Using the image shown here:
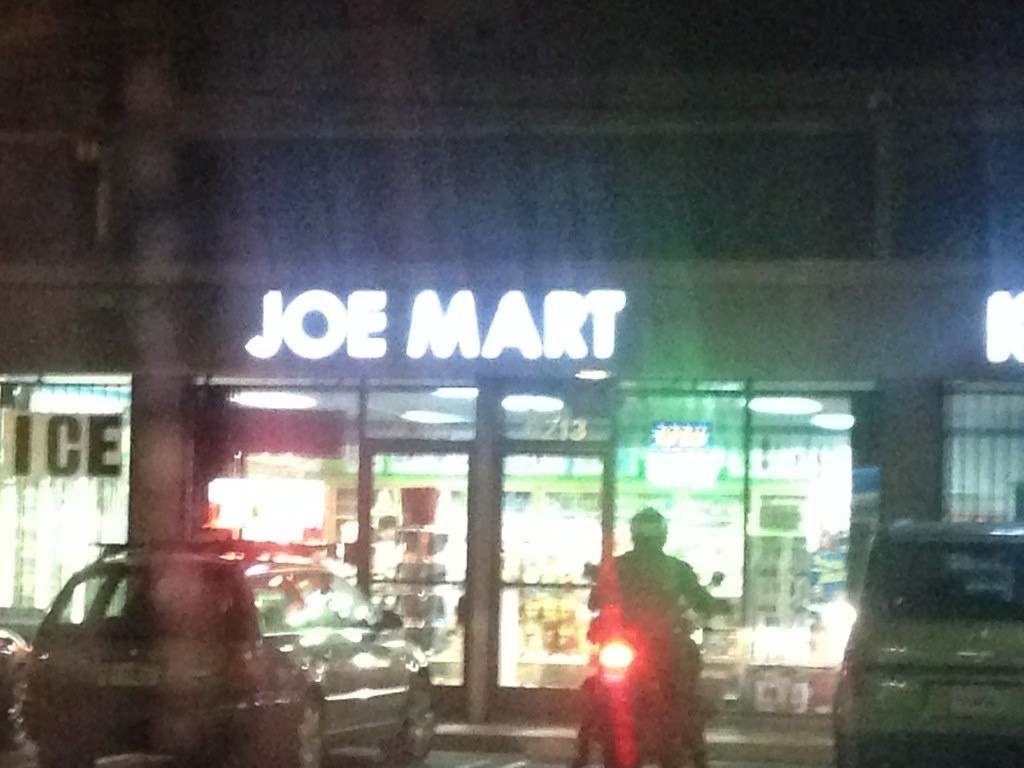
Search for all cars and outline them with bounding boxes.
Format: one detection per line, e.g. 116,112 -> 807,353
0,624 -> 45,757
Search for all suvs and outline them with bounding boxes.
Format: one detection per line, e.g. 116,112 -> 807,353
25,533 -> 443,766
828,519 -> 1024,768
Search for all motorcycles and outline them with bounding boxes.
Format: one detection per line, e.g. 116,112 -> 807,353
579,558 -> 731,768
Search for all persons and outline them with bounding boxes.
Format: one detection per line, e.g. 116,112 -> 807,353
582,507 -> 728,767
